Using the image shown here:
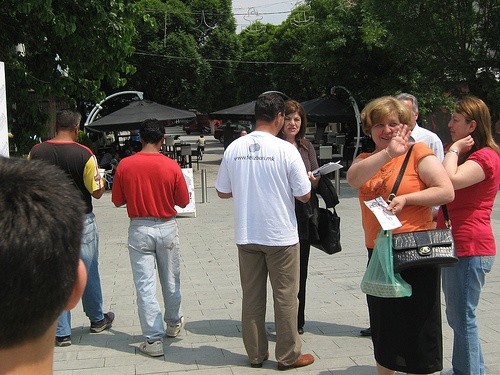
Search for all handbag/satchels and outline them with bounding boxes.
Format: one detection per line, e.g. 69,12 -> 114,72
360,230 -> 412,298
309,207 -> 342,255
392,228 -> 461,273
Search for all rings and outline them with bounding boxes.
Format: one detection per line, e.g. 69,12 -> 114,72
396,133 -> 400,137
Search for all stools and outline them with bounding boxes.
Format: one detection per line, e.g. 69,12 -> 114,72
176,146 -> 191,171
317,144 -> 345,167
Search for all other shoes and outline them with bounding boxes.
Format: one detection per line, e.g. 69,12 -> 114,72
166,315 -> 184,338
360,327 -> 371,337
299,328 -> 304,335
139,340 -> 164,357
55,335 -> 71,347
90,312 -> 115,333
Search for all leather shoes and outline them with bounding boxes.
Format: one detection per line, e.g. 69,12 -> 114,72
250,350 -> 269,368
278,354 -> 314,371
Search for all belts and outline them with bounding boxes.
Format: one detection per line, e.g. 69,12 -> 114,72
131,220 -> 172,229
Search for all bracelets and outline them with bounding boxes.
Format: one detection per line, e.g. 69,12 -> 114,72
448,149 -> 460,157
386,148 -> 394,160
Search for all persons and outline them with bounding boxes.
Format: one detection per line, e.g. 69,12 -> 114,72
100,138 -> 142,176
359,93 -> 446,335
347,97 -> 454,375
276,100 -> 321,334
314,139 -> 333,151
112,119 -> 190,357
26,111 -> 115,347
173,135 -> 185,161
0,157 -> 88,375
224,123 -> 234,150
432,97 -> 500,375
241,129 -> 247,137
216,91 -> 315,370
196,134 -> 206,155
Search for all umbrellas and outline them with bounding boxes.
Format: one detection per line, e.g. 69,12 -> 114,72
301,95 -> 355,121
210,100 -> 255,120
86,100 -> 196,132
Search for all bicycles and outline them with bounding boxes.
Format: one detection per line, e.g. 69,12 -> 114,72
196,142 -> 204,160
